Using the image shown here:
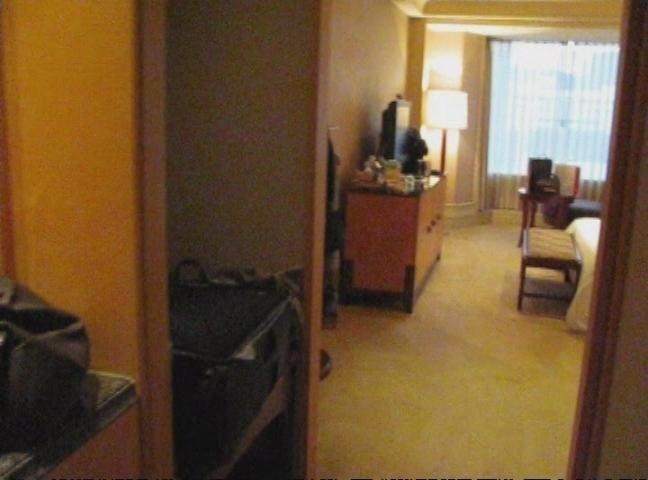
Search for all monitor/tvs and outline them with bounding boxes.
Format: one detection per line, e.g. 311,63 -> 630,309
382,99 -> 411,161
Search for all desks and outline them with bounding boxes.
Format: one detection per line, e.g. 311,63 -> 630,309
518,186 -> 575,249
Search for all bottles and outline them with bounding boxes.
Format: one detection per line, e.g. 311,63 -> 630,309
383,160 -> 401,184
361,155 -> 381,184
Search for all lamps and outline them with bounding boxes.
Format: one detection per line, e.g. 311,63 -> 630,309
424,87 -> 469,176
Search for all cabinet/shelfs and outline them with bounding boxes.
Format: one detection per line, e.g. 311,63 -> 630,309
338,171 -> 447,314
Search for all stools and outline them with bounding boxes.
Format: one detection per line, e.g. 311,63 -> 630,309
516,228 -> 582,311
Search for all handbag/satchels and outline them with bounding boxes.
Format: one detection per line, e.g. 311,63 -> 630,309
0,275 -> 92,454
169,249 -> 309,470
